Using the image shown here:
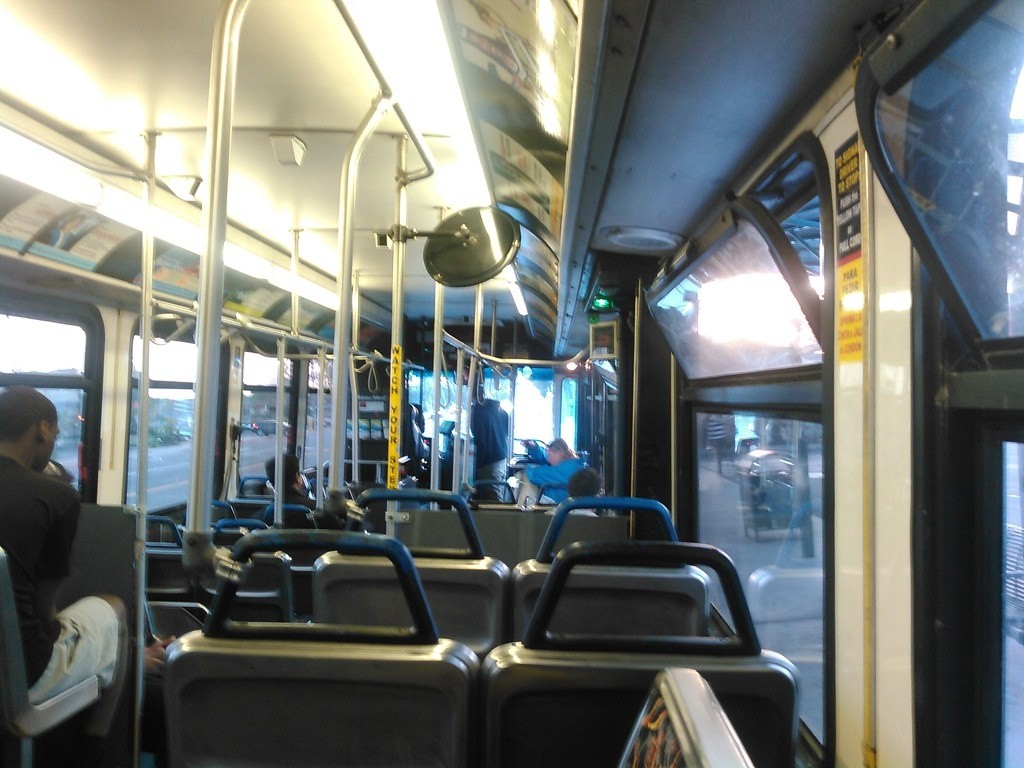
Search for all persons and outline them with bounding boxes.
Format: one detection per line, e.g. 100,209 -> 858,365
0,385 -> 430,768
518,438 -> 600,517
470,386 -> 509,501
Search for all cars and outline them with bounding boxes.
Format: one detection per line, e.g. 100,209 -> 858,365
251,419 -> 290,437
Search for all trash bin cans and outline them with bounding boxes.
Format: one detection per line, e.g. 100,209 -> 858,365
734,449 -> 803,541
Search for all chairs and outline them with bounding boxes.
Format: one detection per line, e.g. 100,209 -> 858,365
0,457 -> 802,768
